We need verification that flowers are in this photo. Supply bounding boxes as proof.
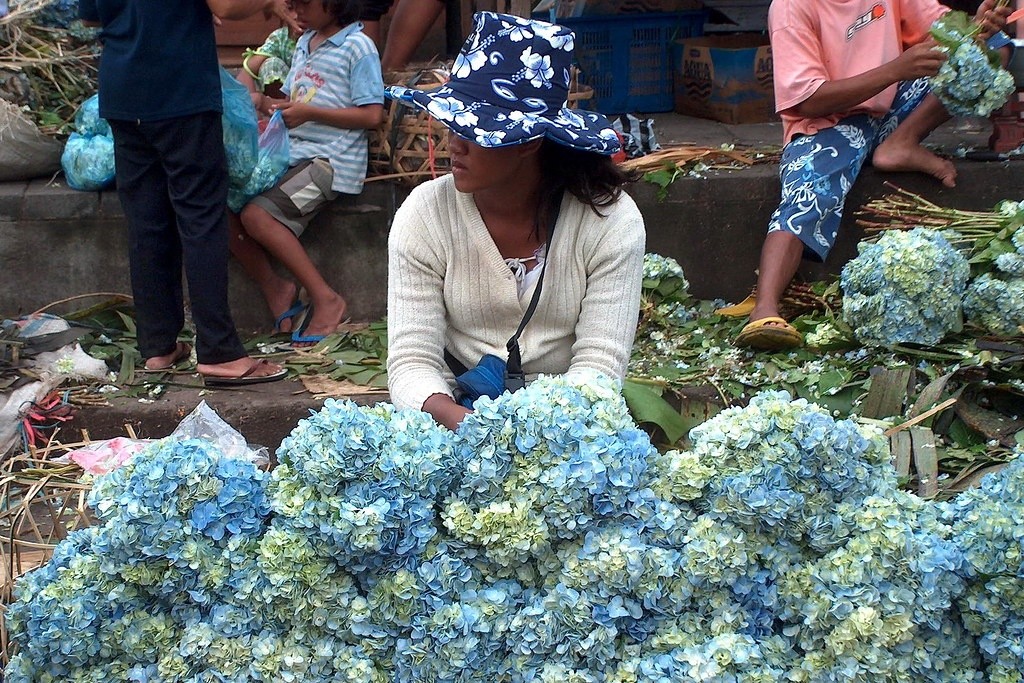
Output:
[839,225,970,347]
[962,272,1024,339]
[927,0,1016,119]
[996,200,1024,277]
[0,374,1024,683]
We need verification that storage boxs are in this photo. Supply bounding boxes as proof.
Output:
[671,32,781,125]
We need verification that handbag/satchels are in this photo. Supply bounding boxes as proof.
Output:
[456,354,526,413]
[612,113,662,159]
[61,64,290,213]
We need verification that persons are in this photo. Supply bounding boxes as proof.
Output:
[384,11,645,432]
[734,0,1017,353]
[75,0,442,388]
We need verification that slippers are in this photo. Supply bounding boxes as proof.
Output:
[144,340,191,374]
[735,317,802,352]
[274,298,314,333]
[289,328,327,351]
[714,293,757,317]
[205,359,288,386]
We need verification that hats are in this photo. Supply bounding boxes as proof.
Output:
[384,10,622,155]
